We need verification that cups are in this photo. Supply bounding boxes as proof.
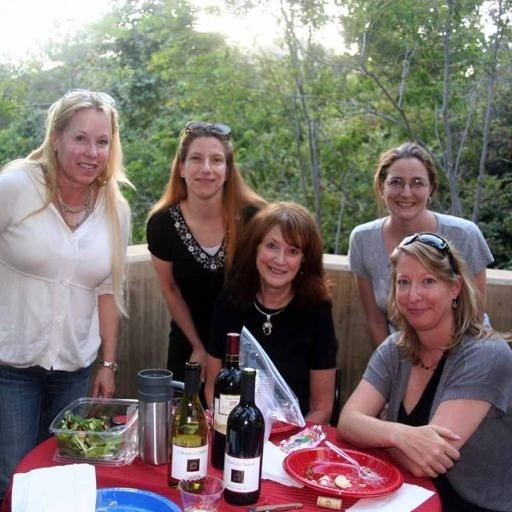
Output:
[137,368,173,465]
[178,474,226,512]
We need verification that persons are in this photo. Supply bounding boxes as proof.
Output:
[337,232,510,507]
[212,203,339,433]
[0,90,132,502]
[147,121,272,407]
[347,145,494,349]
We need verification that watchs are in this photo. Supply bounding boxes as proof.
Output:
[97,359,117,373]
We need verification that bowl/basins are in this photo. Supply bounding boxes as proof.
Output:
[49,397,139,459]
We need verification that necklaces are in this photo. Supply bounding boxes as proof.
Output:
[252,299,291,336]
[408,350,446,372]
[50,176,96,229]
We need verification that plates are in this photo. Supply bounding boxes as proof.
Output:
[281,447,404,498]
[95,487,183,512]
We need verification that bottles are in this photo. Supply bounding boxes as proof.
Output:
[167,361,209,494]
[211,333,243,470]
[223,367,266,506]
[137,368,185,466]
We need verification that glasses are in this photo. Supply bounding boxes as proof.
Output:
[183,118,232,138]
[384,175,433,193]
[399,231,461,276]
[63,88,117,106]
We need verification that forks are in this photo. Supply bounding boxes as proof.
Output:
[324,440,383,481]
